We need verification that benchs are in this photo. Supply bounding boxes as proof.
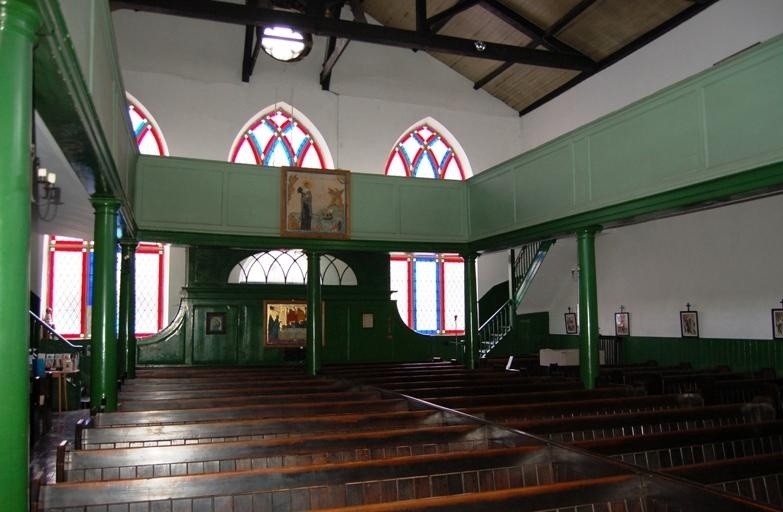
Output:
[29,361,781,511]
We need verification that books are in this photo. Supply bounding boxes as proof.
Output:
[27,352,78,372]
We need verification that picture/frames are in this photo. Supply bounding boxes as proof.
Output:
[262,299,324,347]
[563,313,577,334]
[771,308,783,341]
[205,310,226,334]
[612,312,631,337]
[679,310,699,339]
[279,166,350,238]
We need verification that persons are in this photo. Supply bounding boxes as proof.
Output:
[213,317,222,331]
[616,314,627,334]
[267,306,282,342]
[685,316,694,335]
[777,317,783,336]
[43,307,55,336]
[567,319,575,332]
[299,181,312,231]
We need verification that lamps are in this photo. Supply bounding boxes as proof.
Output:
[30,56,62,221]
